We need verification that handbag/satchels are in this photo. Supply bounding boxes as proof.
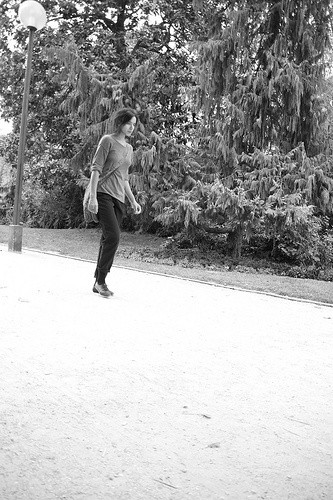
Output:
[83,183,99,223]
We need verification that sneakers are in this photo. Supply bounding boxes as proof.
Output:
[93,281,114,298]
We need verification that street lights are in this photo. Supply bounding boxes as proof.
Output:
[7,0,49,252]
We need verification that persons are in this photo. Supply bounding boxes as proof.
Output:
[88,109,142,298]
